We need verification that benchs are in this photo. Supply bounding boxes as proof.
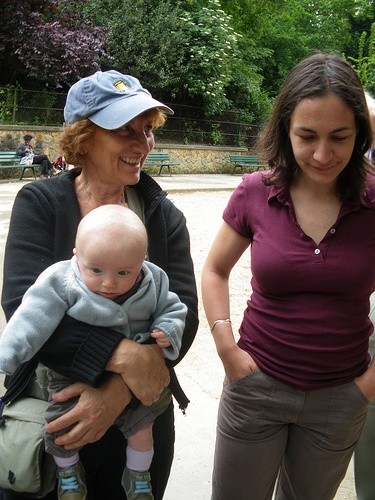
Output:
[0,151,42,180]
[229,154,269,176]
[145,153,179,177]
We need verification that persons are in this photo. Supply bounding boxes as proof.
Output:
[0,205,189,500]
[15,134,63,180]
[355,93,375,500]
[0,69,200,500]
[200,52,375,500]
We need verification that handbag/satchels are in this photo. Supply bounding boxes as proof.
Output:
[0,396,58,499]
[20,156,33,165]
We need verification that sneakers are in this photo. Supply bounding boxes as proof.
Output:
[122,466,154,500]
[55,461,88,500]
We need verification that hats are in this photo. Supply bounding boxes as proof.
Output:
[24,135,32,140]
[64,70,174,130]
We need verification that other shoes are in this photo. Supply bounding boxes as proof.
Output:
[53,170,61,175]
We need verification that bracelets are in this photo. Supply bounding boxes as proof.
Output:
[211,318,232,332]
